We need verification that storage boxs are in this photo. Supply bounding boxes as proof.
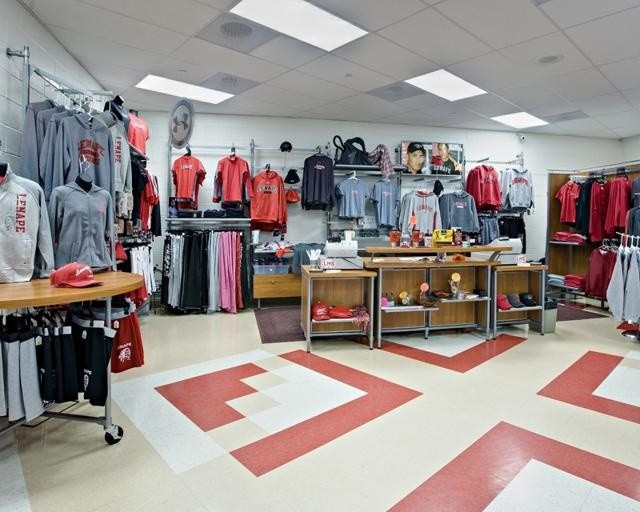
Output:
[252,263,290,274]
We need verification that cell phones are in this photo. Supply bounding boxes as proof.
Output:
[309,269,324,273]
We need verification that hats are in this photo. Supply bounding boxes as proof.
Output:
[49,261,105,288]
[407,142,425,154]
[312,300,329,320]
[280,142,292,152]
[497,292,537,309]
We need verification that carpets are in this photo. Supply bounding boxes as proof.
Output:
[251,300,612,344]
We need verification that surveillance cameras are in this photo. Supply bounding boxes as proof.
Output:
[519,136,525,140]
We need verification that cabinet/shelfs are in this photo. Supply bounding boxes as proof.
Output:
[295,246,549,353]
[253,273,302,308]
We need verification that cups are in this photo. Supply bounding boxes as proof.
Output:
[390,229,401,242]
[412,230,424,242]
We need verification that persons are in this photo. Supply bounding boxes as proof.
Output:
[403,143,463,174]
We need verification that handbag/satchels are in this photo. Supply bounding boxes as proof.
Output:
[332,136,371,165]
[284,169,300,183]
[329,305,352,318]
[286,188,301,204]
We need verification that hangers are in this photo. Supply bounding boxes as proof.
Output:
[598,238,611,250]
[617,233,640,250]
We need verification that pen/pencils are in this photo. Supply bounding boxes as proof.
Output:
[305,249,321,256]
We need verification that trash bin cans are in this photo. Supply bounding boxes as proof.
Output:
[529,297,558,332]
